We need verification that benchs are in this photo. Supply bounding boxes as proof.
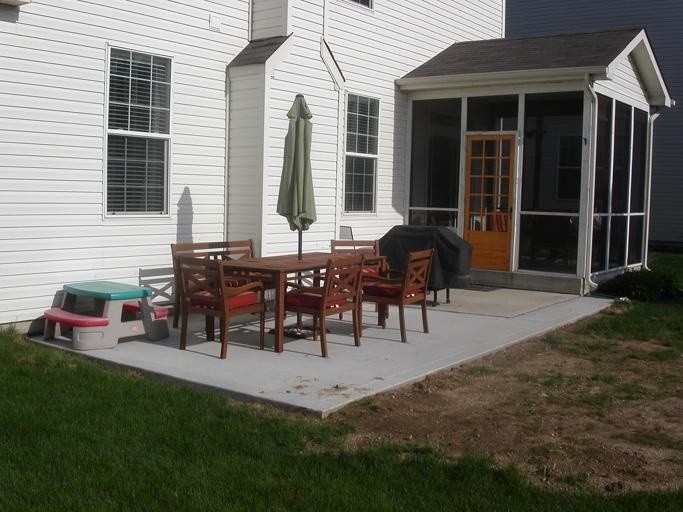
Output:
[43,301,173,350]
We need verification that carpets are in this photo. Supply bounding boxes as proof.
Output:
[404,283,580,318]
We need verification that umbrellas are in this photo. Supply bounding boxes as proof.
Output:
[276,94,317,260]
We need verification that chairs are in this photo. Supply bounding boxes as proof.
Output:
[171,239,434,358]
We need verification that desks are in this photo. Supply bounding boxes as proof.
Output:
[61,281,152,351]
